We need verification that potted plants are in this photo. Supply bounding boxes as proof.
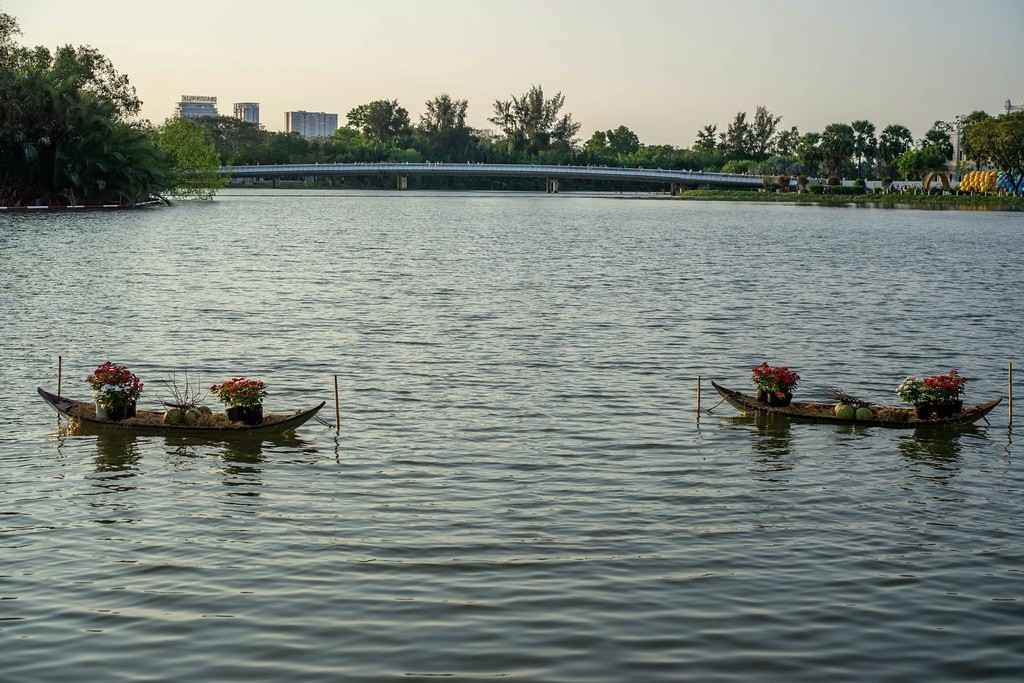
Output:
[796,177,809,194]
[776,176,790,194]
[758,175,770,193]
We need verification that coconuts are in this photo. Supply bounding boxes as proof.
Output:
[164,406,212,426]
[834,402,874,420]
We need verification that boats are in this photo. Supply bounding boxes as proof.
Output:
[37,387,326,434]
[711,381,1003,427]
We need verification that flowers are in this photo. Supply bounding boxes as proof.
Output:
[896,376,931,402]
[210,378,245,407]
[765,366,801,399]
[750,362,773,391]
[85,361,129,409]
[925,376,957,403]
[124,371,144,405]
[924,377,944,403]
[230,379,266,405]
[946,370,967,401]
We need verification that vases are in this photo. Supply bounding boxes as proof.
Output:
[123,400,137,419]
[767,392,793,408]
[952,400,963,413]
[915,402,932,420]
[224,406,244,423]
[937,404,954,419]
[932,402,939,413]
[757,387,768,401]
[243,405,264,425]
[95,401,123,422]
